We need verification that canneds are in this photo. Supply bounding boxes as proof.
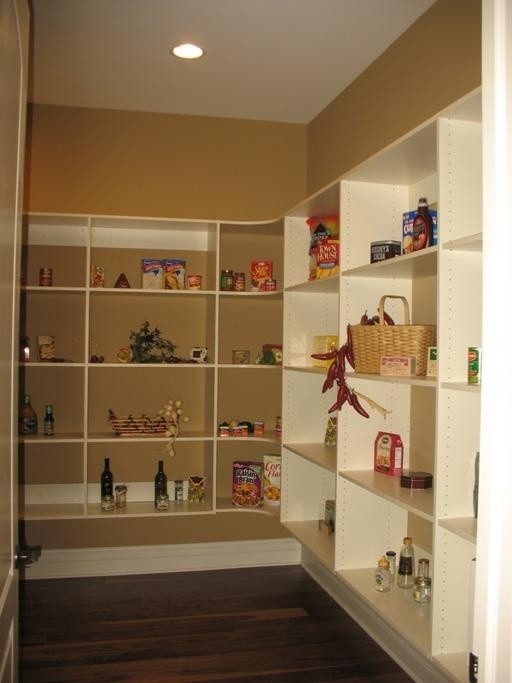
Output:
[254,421,264,436]
[275,417,282,437]
[232,425,249,437]
[468,348,481,384]
[221,270,244,291]
[39,266,53,287]
[370,241,401,264]
[187,274,202,290]
[265,279,276,291]
[230,349,250,364]
[219,427,229,436]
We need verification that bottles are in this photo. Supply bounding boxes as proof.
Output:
[45,405,55,437]
[18,394,38,436]
[373,535,432,606]
[411,197,434,253]
[221,269,234,291]
[102,493,115,512]
[154,459,168,508]
[18,335,32,363]
[274,416,282,440]
[233,272,246,292]
[37,266,53,286]
[100,457,112,502]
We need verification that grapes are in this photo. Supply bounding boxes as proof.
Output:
[90,354,105,364]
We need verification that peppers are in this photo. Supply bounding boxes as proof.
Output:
[311,306,395,418]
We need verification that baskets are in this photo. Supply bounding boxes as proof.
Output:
[106,413,181,437]
[348,294,437,377]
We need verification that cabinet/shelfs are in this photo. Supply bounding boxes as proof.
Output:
[280,87,486,683]
[10,215,284,520]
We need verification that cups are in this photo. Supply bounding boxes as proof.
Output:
[156,493,169,511]
[115,485,128,508]
